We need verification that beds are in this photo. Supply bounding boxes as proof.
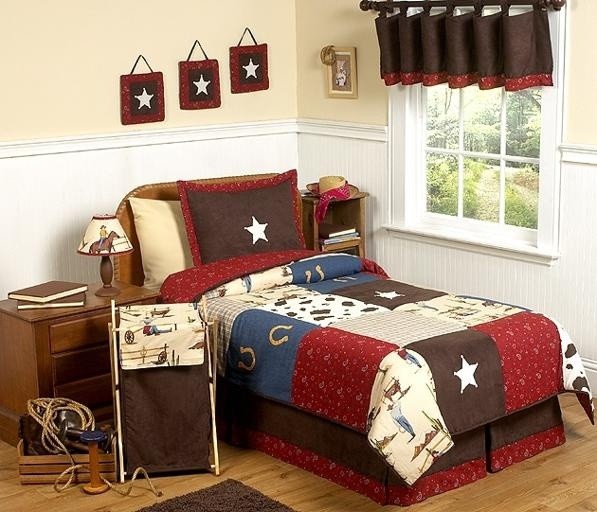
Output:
[113,174,595,506]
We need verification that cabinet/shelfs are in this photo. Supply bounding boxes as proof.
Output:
[301,191,386,260]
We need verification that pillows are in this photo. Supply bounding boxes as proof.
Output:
[127,192,193,289]
[174,168,312,267]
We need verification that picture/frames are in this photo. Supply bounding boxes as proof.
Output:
[228,43,270,95]
[319,42,358,100]
[176,57,221,111]
[115,71,166,128]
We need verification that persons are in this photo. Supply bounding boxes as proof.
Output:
[97,225,109,253]
[143,317,172,336]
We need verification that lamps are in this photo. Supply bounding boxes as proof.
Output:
[74,213,134,297]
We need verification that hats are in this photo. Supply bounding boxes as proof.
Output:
[307,175,358,200]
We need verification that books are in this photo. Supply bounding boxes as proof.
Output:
[317,223,360,245]
[7,280,88,311]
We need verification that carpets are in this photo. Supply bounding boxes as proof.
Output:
[134,476,298,512]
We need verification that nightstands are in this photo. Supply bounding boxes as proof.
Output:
[0,279,160,451]
